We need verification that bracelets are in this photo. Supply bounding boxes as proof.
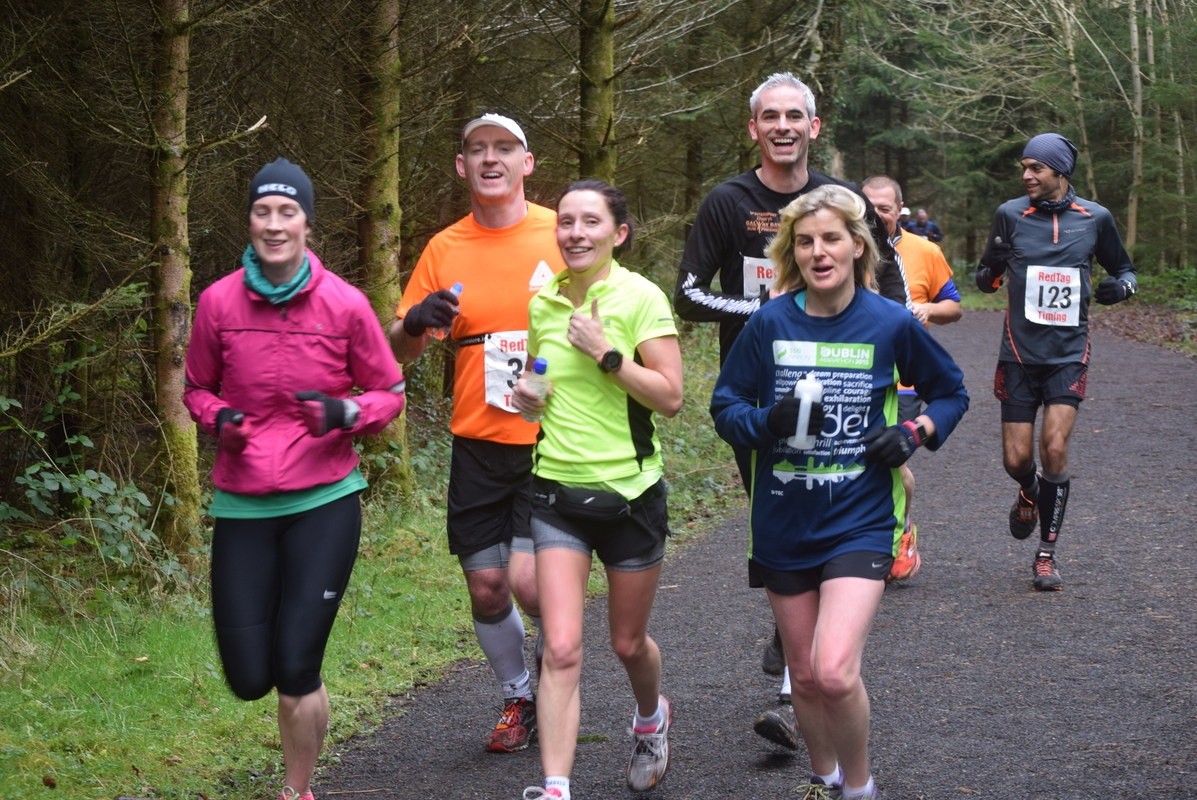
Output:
[902,421,923,449]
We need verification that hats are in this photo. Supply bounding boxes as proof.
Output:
[250,157,314,219]
[463,113,528,151]
[1022,133,1078,178]
[900,208,910,216]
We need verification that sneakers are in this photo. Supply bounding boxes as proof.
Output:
[280,787,313,800]
[761,627,785,674]
[890,523,921,580]
[753,704,801,750]
[838,783,881,800]
[1033,558,1064,590]
[487,693,537,752]
[795,768,844,800]
[522,786,570,800]
[535,630,544,660]
[626,694,673,791]
[1010,472,1043,539]
[779,667,792,701]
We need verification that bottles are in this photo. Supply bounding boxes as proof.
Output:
[786,374,823,450]
[519,357,548,423]
[425,283,463,347]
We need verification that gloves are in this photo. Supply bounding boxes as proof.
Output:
[216,409,248,454]
[987,236,1014,276]
[928,232,934,238]
[768,373,825,438]
[295,391,359,438]
[862,419,930,470]
[1094,278,1128,305]
[403,290,459,336]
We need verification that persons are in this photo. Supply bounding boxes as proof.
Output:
[675,72,911,751]
[975,130,1139,591]
[513,179,674,800]
[709,184,970,800]
[861,174,963,583]
[181,156,408,800]
[387,113,567,752]
[896,207,944,244]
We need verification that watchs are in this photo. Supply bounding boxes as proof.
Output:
[596,345,623,374]
[344,397,362,428]
[910,418,928,446]
